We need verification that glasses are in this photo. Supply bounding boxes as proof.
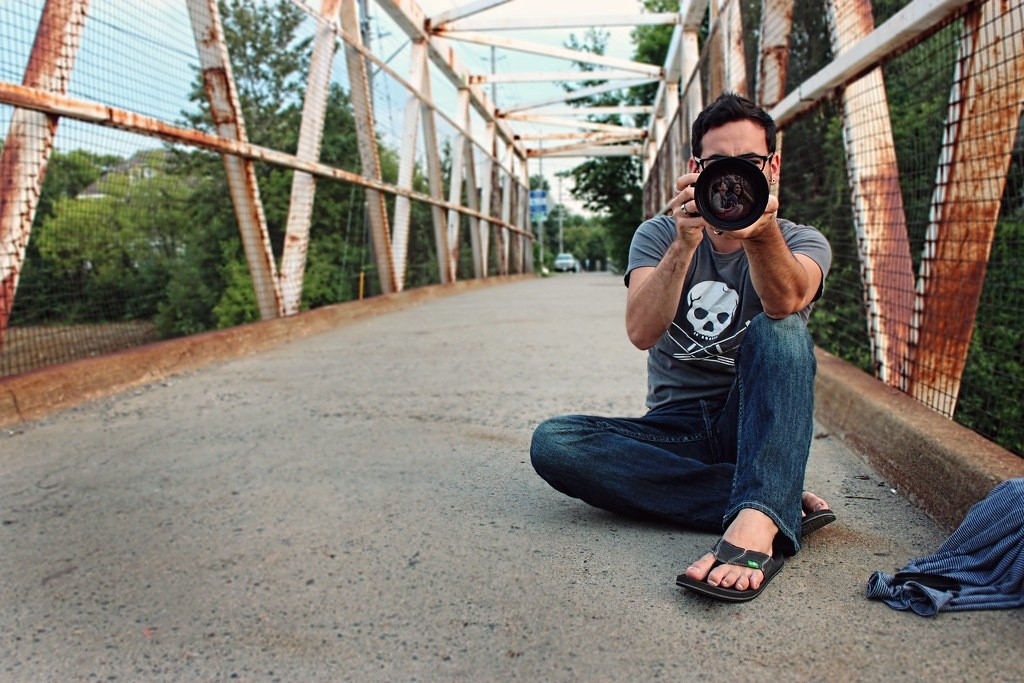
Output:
[694,153,775,171]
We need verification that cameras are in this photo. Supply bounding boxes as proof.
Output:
[687,156,769,231]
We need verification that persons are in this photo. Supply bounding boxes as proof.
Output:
[711,181,744,218]
[530,91,837,602]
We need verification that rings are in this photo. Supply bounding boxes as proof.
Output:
[713,230,724,236]
[681,204,688,214]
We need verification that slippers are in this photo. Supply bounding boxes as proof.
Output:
[676,538,785,602]
[802,490,836,538]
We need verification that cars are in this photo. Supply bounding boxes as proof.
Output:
[554,253,580,273]
